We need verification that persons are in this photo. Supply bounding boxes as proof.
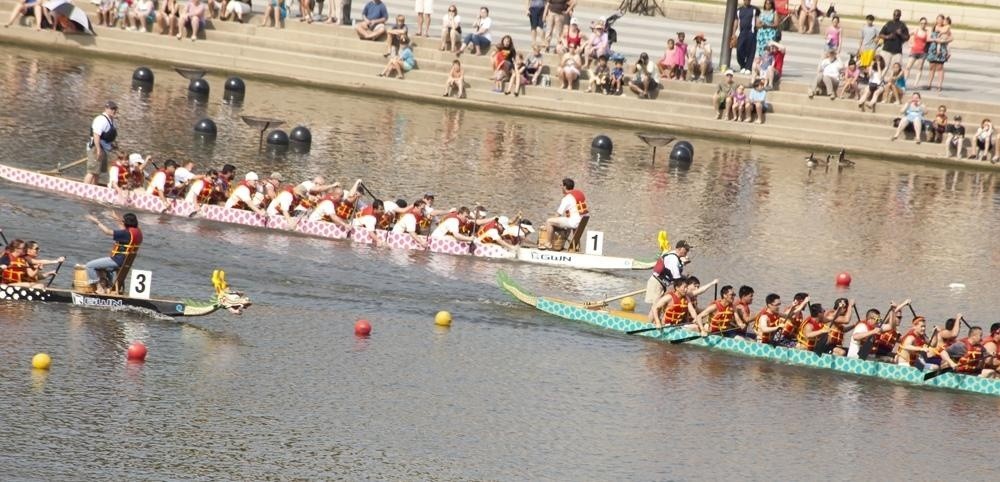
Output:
[105,153,534,253]
[732,1,952,109]
[259,0,388,40]
[84,212,142,297]
[491,1,660,98]
[646,241,691,323]
[378,0,491,99]
[1,239,24,284]
[2,1,253,40]
[892,93,994,160]
[657,31,712,82]
[86,101,119,185]
[25,243,67,281]
[651,275,1000,377]
[538,178,588,251]
[713,70,768,124]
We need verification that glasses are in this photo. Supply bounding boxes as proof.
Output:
[209,175,218,181]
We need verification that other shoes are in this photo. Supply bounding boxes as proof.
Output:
[440,45,483,57]
[715,111,762,124]
[300,17,345,25]
[379,52,403,78]
[661,71,707,83]
[424,33,428,37]
[493,77,650,98]
[809,75,941,144]
[457,96,462,98]
[443,93,448,97]
[741,68,773,91]
[416,32,420,35]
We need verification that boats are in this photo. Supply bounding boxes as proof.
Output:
[0,269,253,319]
[494,266,999,397]
[0,155,661,274]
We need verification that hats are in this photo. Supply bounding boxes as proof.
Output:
[271,171,286,182]
[725,69,733,76]
[129,154,145,163]
[520,223,535,232]
[105,101,118,109]
[400,38,409,43]
[165,159,180,168]
[293,184,308,199]
[499,216,509,231]
[676,240,693,249]
[244,171,259,181]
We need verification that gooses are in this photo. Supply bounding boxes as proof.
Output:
[837,147,856,167]
[817,153,835,167]
[804,151,817,168]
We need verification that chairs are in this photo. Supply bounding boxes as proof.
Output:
[103,251,136,294]
[564,214,590,251]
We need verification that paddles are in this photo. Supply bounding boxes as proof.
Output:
[626,323,695,335]
[961,316,971,329]
[385,207,525,261]
[585,282,717,309]
[138,158,223,219]
[774,301,929,362]
[924,354,992,381]
[258,178,380,241]
[670,326,741,345]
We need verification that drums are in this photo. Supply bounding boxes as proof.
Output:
[74,265,98,296]
[539,226,567,252]
